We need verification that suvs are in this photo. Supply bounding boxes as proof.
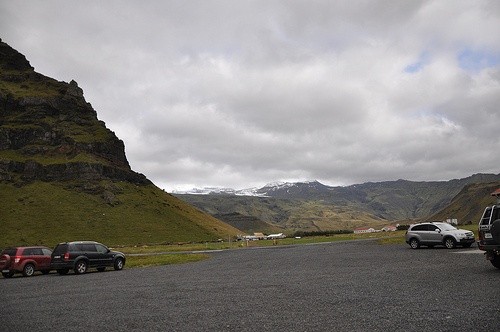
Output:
[48,240,126,275]
[0,246,54,278]
[405,221,475,250]
[478,204,500,270]
